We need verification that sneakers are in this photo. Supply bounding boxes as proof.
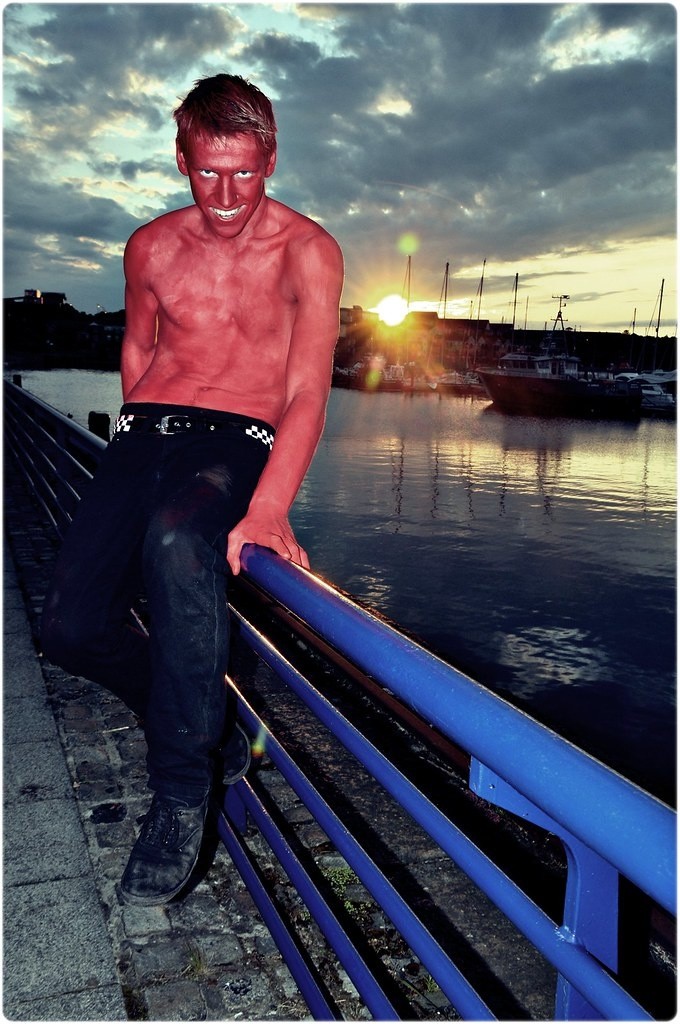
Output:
[215,717,250,788]
[119,795,207,910]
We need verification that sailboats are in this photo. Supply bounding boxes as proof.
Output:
[333,256,676,419]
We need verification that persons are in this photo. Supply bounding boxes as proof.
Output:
[61,68,344,907]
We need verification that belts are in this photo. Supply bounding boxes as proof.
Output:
[112,414,277,454]
